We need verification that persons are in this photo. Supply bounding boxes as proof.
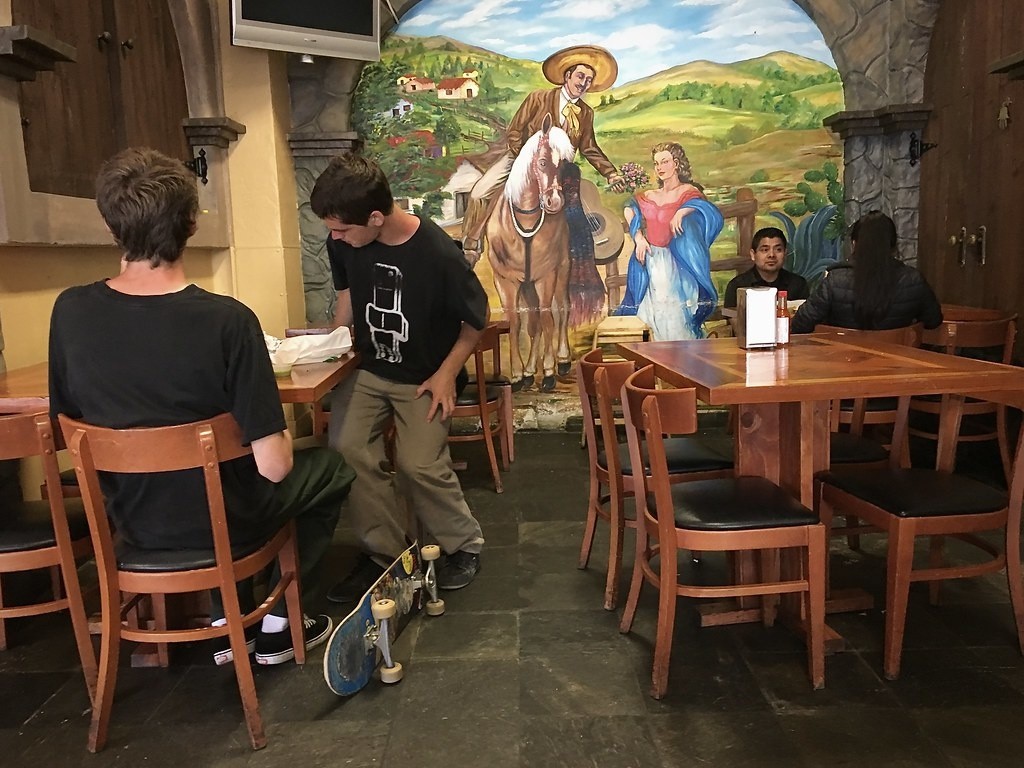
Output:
[791,209,946,335]
[723,226,813,342]
[311,147,492,609]
[48,149,356,668]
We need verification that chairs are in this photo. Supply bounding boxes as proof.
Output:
[617,363,828,699]
[387,322,509,495]
[572,349,733,612]
[900,313,1018,487]
[53,412,307,754]
[814,324,922,550]
[462,322,516,471]
[814,397,1023,683]
[1,405,144,714]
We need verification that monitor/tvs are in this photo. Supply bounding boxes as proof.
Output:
[229,0,380,62]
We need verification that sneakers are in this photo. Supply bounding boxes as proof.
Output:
[211,612,258,665]
[438,549,481,589]
[255,615,333,665]
[327,562,384,603]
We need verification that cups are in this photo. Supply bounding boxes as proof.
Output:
[272,364,293,378]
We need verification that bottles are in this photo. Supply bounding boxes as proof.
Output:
[775,291,792,347]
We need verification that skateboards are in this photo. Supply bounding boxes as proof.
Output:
[322,536,447,697]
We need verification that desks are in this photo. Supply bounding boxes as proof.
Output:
[285,327,459,475]
[720,303,1004,337]
[0,338,360,635]
[616,331,1024,628]
